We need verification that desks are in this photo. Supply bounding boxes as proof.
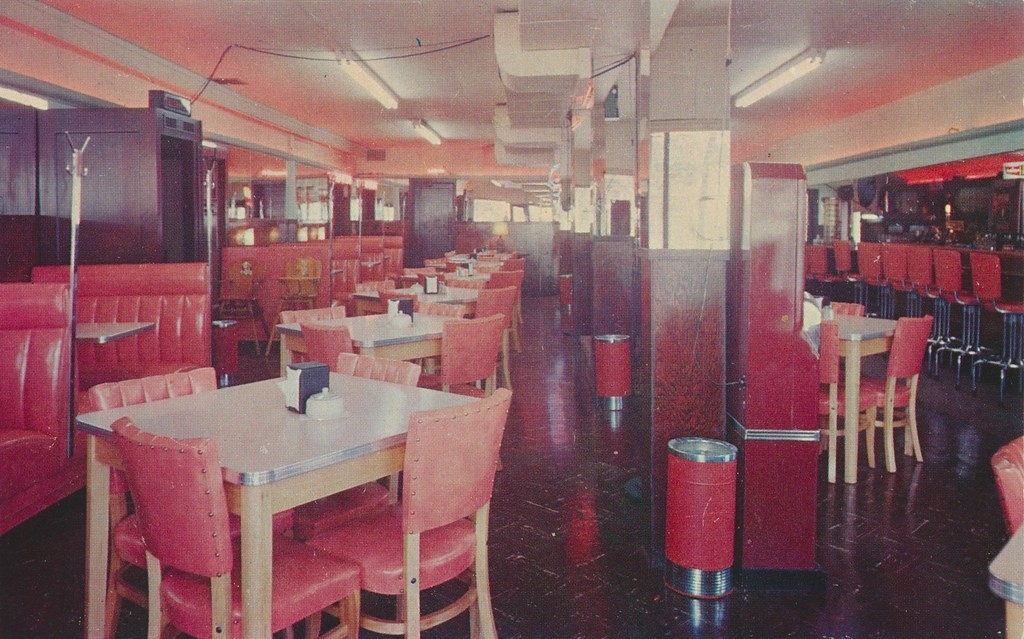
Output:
[400,273,490,289]
[448,253,512,263]
[75,370,488,639]
[988,525,1024,639]
[427,261,504,274]
[837,315,899,483]
[352,287,486,313]
[275,311,468,378]
[72,322,155,344]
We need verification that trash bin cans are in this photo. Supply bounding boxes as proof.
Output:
[594,335,631,411]
[664,436,738,599]
[559,275,573,335]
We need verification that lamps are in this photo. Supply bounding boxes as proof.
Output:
[335,49,398,110]
[730,46,827,109]
[412,120,442,146]
[492,222,509,254]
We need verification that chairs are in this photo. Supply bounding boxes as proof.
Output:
[87,246,529,639]
[809,301,1024,538]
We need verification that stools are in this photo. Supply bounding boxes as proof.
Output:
[805,233,1024,405]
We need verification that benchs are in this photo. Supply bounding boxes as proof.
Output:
[221,231,405,342]
[1,236,213,534]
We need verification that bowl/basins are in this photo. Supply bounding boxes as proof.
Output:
[410,283,424,294]
[389,310,413,329]
[304,387,348,420]
